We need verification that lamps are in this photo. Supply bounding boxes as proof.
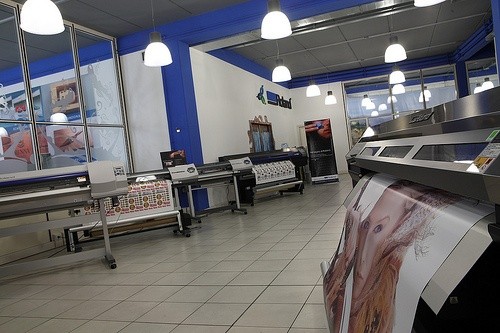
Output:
[272,40,292,82]
[325,72,337,105]
[474,65,484,94]
[144,0,173,67]
[261,0,292,40]
[482,69,494,90]
[385,14,407,63]
[19,0,65,35]
[306,80,321,98]
[362,63,431,117]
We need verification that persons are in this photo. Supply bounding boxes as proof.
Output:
[304,118,331,138]
[323,179,461,333]
